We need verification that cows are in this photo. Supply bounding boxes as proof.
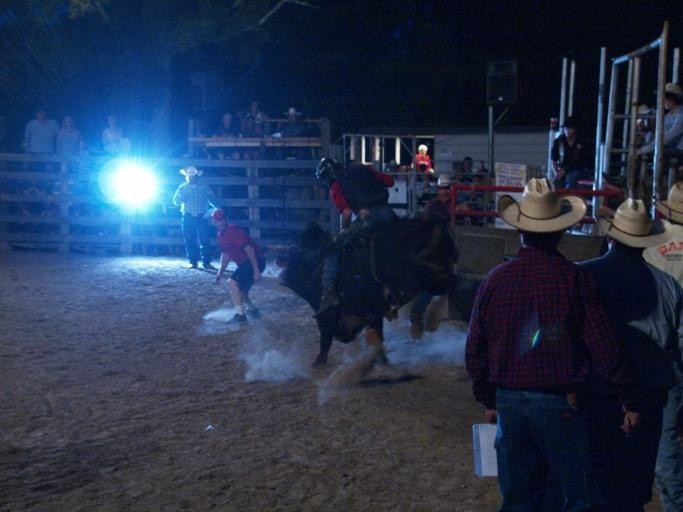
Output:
[275,213,462,372]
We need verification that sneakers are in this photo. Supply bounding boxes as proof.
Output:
[223,314,247,325]
[188,262,213,270]
[245,308,260,320]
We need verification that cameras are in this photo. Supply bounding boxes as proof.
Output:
[550,175,566,184]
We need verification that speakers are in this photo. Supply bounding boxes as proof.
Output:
[485,59,518,106]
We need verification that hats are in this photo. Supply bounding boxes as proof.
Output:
[179,166,204,176]
[496,177,588,233]
[428,174,457,187]
[283,107,303,116]
[654,181,683,224]
[596,197,672,249]
[561,116,579,128]
[214,212,226,221]
[636,104,652,114]
[653,82,682,96]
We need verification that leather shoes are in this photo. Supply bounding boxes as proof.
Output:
[311,289,341,318]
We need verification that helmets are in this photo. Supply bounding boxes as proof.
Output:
[315,156,342,184]
